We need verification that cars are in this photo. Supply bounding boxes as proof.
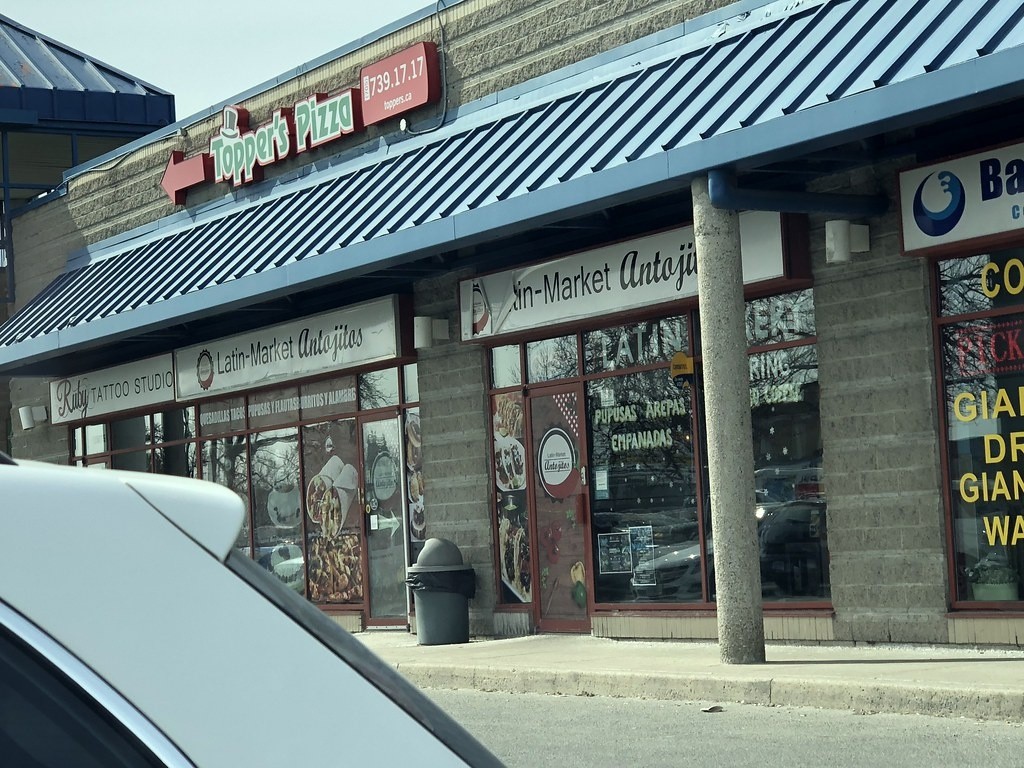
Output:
[0,452,505,768]
[635,467,823,601]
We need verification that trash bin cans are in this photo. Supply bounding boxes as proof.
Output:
[406,538,474,644]
